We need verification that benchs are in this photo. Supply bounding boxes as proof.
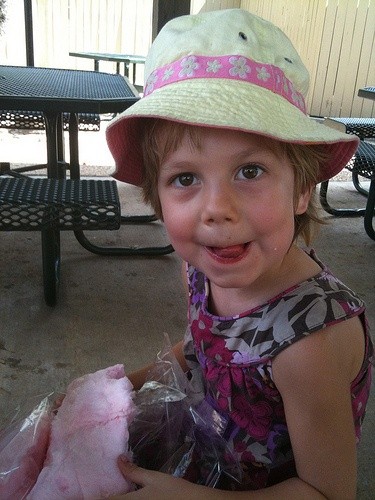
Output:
[0,178,121,306]
[325,117,375,183]
[345,140,375,226]
[0,110,100,132]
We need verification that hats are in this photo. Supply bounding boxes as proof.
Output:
[105,8,360,189]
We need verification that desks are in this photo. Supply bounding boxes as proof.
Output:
[0,64,142,179]
[69,52,146,85]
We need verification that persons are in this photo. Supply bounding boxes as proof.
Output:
[55,8,375,500]
